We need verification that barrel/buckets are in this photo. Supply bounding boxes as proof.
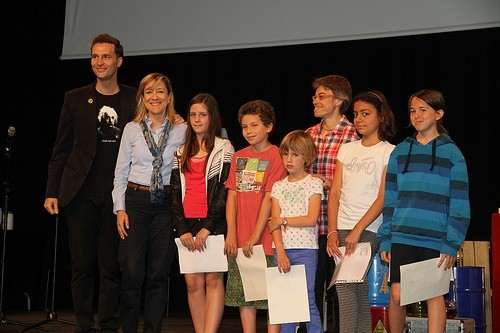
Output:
[445,266,486,333]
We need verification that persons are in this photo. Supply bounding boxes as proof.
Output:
[169,92,236,333]
[377,90,470,333]
[44,35,139,333]
[326,93,397,333]
[299,76,360,333]
[224,100,287,333]
[270,129,328,333]
[111,73,188,333]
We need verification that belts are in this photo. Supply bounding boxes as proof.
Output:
[127,182,171,193]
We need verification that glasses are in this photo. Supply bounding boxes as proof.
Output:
[312,93,333,101]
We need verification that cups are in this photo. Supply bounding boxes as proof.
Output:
[446,299,457,320]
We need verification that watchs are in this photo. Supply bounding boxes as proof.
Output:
[282,217,287,228]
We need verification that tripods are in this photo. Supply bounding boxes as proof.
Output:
[0,151,76,333]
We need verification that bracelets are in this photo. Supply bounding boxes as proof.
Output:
[327,230,338,240]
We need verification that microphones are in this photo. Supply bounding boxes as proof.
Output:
[5,126,15,151]
